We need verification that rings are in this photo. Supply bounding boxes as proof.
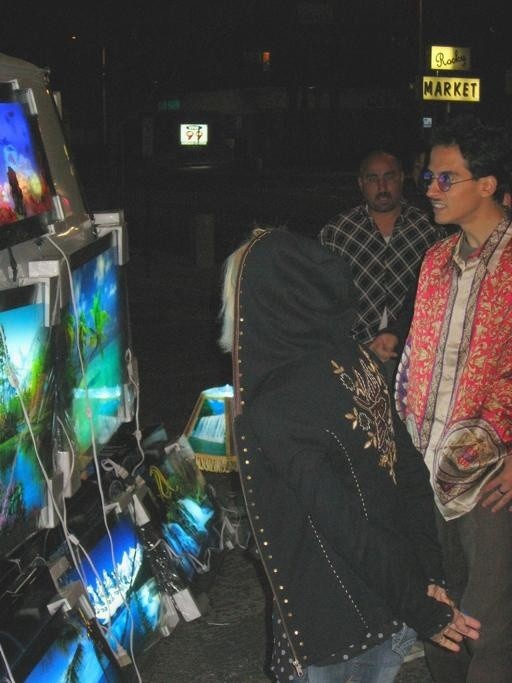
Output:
[498,489,505,497]
[440,639,443,645]
[444,628,451,637]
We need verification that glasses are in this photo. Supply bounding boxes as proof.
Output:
[423,170,473,192]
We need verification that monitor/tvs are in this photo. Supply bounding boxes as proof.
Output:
[178,121,212,148]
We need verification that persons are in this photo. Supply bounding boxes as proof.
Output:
[315,147,448,344]
[367,166,512,360]
[216,225,482,683]
[392,120,512,682]
